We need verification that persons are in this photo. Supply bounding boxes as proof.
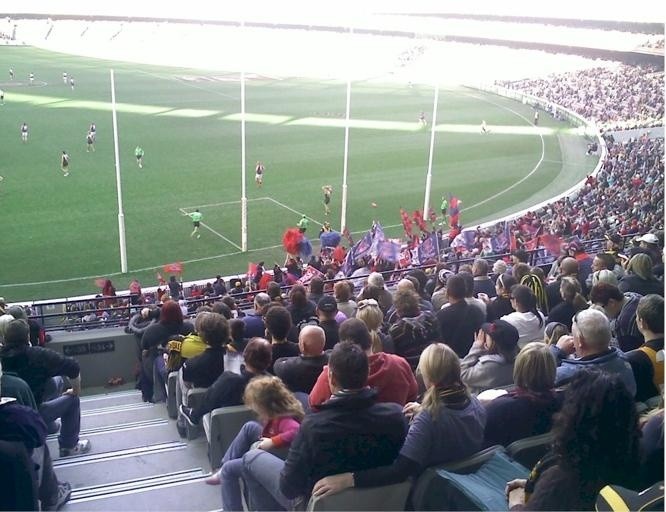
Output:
[481,120,487,135]
[86,124,96,152]
[418,111,428,127]
[124,132,665,512]
[61,151,71,177]
[9,67,76,90]
[494,32,663,132]
[66,279,118,328]
[21,123,28,142]
[1,16,19,47]
[1,296,90,512]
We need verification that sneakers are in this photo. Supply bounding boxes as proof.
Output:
[58,439,91,457]
[206,472,221,486]
[41,479,72,512]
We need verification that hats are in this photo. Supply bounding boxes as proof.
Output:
[635,233,659,245]
[481,320,520,347]
[317,295,337,313]
